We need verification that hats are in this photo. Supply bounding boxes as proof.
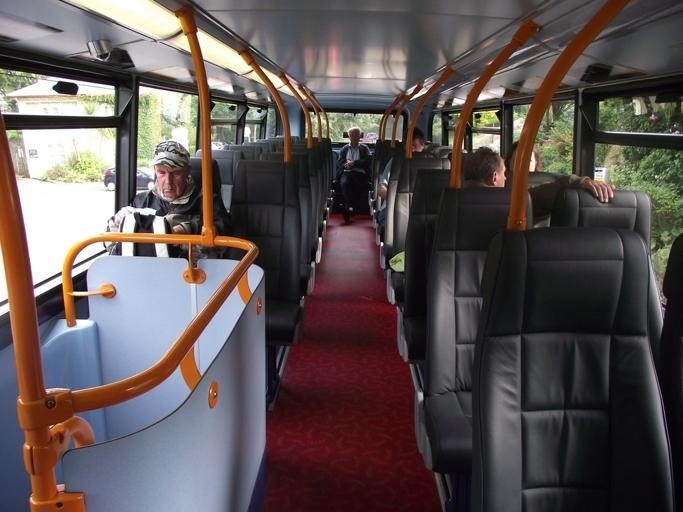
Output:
[149,140,190,170]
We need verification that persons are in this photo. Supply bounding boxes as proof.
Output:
[376,126,424,241]
[503,140,543,173]
[460,146,616,227]
[102,139,233,259]
[335,127,373,224]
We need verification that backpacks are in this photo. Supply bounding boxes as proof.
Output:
[108,206,183,258]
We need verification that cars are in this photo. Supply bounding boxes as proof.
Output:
[104,163,156,192]
